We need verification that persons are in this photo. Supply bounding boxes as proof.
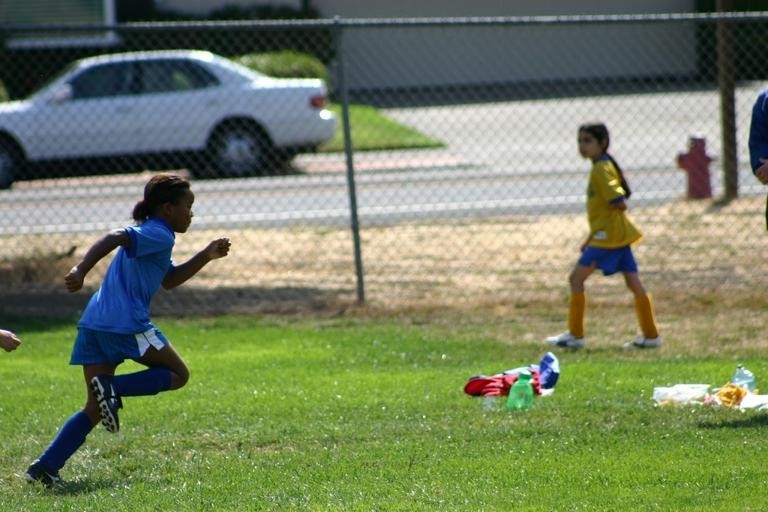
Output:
[27,175,231,489]
[544,124,661,350]
[749,88,768,230]
[0,329,21,352]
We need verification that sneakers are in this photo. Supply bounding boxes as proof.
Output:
[25,462,60,488]
[89,375,120,433]
[625,336,661,350]
[546,331,584,348]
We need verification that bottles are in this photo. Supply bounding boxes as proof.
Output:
[508,373,532,414]
[735,363,756,394]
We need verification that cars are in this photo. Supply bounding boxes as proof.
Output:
[0,48,338,189]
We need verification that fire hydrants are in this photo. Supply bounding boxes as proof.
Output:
[677,135,715,201]
